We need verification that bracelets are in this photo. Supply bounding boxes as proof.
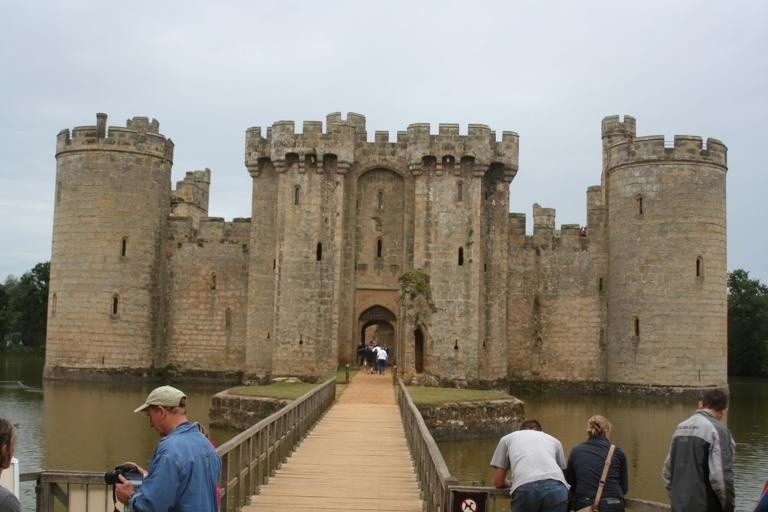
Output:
[126,493,133,504]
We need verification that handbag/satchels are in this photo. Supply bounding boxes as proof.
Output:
[570,505,598,512]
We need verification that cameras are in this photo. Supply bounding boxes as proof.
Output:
[104,465,143,488]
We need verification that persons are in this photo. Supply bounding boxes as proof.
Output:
[200,428,222,509]
[358,339,390,375]
[489,420,572,511]
[114,386,223,512]
[662,389,735,510]
[564,414,629,512]
[0,417,23,512]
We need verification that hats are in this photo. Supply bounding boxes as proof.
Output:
[133,385,188,413]
